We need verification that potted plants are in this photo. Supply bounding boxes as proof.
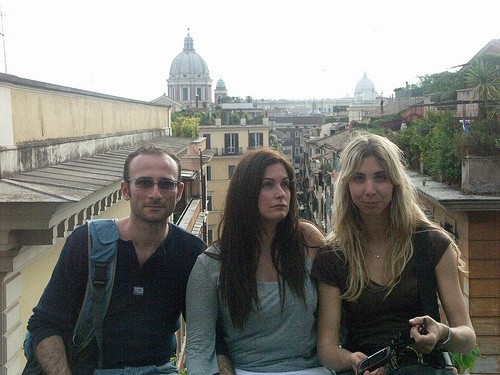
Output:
[394,108,500,194]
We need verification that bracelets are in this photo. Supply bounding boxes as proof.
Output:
[436,326,451,347]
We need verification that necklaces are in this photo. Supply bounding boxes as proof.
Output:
[368,246,387,258]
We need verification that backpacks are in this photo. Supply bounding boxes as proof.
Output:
[21,218,117,375]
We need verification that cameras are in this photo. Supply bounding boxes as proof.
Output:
[357,346,392,375]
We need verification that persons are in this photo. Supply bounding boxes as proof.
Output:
[310,132,476,375]
[294,176,334,220]
[185,148,337,375]
[21,144,236,375]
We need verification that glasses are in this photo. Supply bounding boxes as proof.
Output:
[126,176,180,192]
[389,318,426,353]
